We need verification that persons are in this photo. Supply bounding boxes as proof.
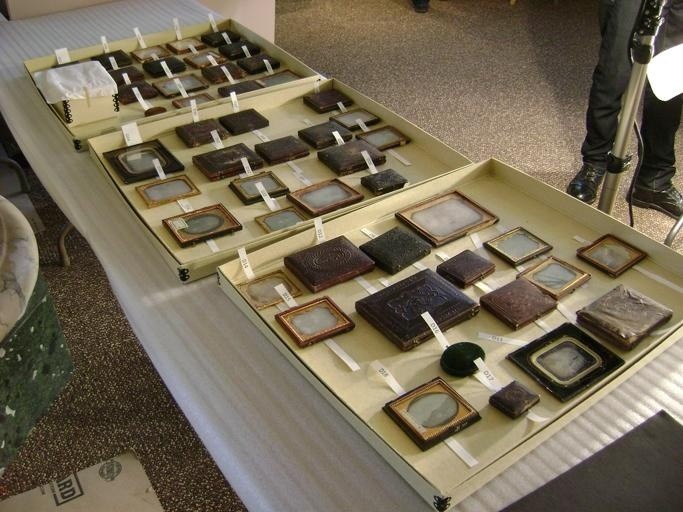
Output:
[565,1,682,221]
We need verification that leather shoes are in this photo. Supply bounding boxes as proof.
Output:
[628,184,683,220]
[567,163,605,205]
